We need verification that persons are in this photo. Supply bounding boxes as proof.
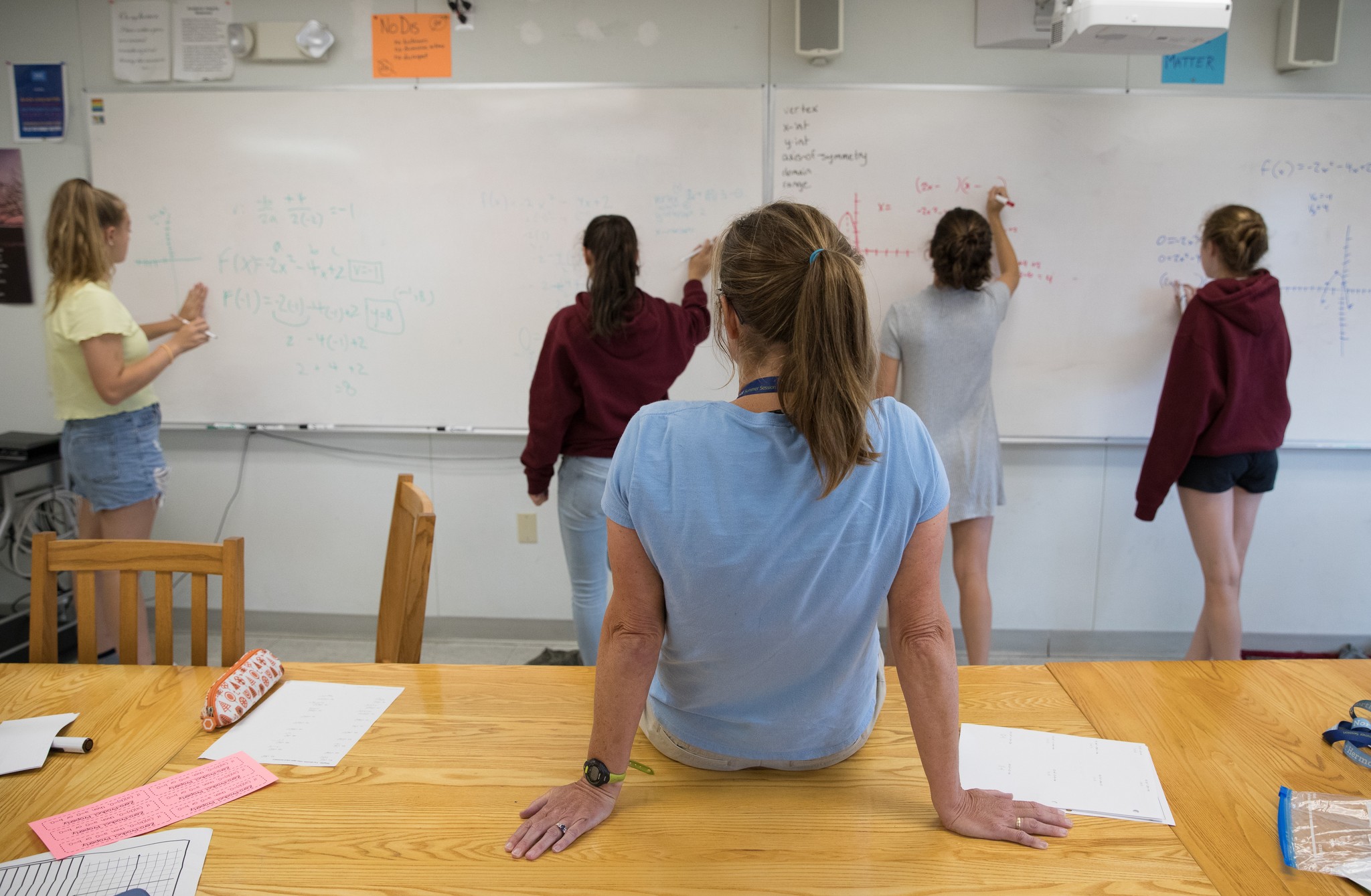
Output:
[502,201,1076,864]
[873,184,1022,664]
[1133,203,1295,661]
[517,213,716,665]
[38,178,214,663]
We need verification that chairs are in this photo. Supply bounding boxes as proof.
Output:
[30,531,245,667]
[375,474,436,663]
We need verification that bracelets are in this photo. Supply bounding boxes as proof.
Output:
[160,343,174,365]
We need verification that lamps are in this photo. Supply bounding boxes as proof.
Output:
[226,17,334,67]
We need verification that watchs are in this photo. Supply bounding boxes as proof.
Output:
[583,758,655,787]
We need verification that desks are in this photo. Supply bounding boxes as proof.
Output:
[1047,658,1371,896]
[0,662,233,896]
[110,664,1220,896]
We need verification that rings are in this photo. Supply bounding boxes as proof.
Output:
[555,823,568,834]
[1016,817,1021,831]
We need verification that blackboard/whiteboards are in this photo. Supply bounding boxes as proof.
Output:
[85,87,767,436]
[767,79,1371,446]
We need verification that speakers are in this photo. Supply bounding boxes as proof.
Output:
[795,0,844,60]
[1277,0,1344,68]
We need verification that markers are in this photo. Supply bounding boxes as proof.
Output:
[169,312,218,341]
[1179,284,1187,316]
[49,735,94,756]
[298,423,336,431]
[679,241,715,265]
[437,425,472,434]
[206,422,246,430]
[994,193,1015,209]
[247,424,285,432]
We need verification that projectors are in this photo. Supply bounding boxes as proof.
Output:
[1049,1,1233,56]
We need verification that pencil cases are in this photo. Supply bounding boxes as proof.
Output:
[196,648,286,736]
